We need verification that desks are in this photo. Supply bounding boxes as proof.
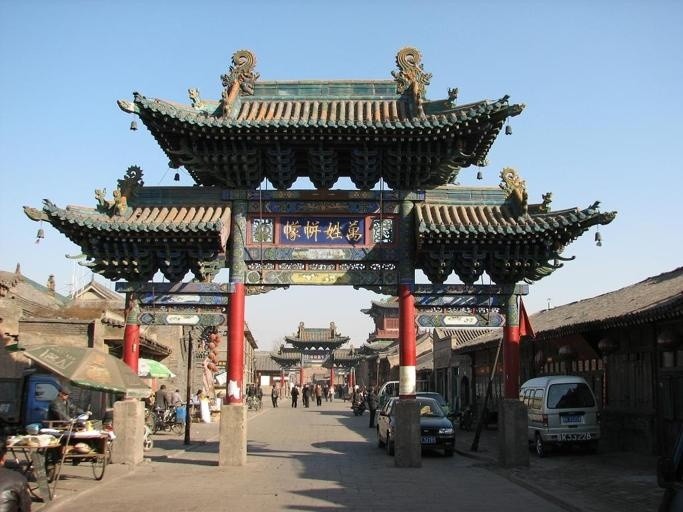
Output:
[5,442,62,504]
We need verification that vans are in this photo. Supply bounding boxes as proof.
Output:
[378,379,428,408]
[518,376,601,457]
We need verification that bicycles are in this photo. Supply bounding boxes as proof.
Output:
[246,394,263,410]
[144,406,182,434]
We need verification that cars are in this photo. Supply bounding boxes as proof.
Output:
[416,391,449,415]
[376,396,455,457]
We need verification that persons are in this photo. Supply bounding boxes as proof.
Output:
[1,428,31,512]
[47,386,92,427]
[141,385,182,428]
[249,383,379,428]
[195,390,203,401]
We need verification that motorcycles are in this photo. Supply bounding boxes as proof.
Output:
[106,420,154,452]
[351,396,366,416]
[458,406,473,432]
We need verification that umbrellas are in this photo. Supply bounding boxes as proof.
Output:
[21,344,153,500]
[138,358,176,379]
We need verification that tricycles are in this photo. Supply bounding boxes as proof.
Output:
[39,402,108,482]
